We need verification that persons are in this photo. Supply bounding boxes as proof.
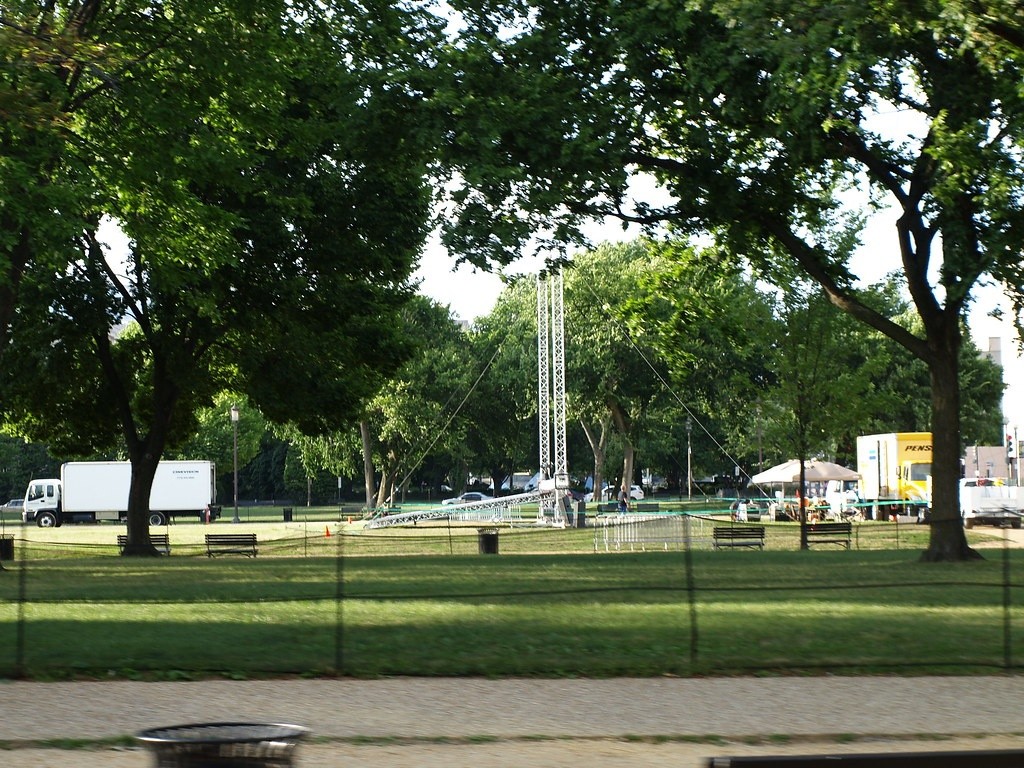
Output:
[819,487,823,497]
[618,485,628,514]
[470,474,475,485]
[820,500,828,520]
[852,486,858,494]
[730,498,742,522]
[784,505,797,522]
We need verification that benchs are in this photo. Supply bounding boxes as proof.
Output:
[799,523,852,549]
[368,508,401,518]
[828,539,830,539]
[340,507,364,520]
[204,533,258,558]
[712,526,765,551]
[637,503,659,512]
[597,505,617,514]
[117,534,171,556]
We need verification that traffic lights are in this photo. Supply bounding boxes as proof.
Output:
[1006,435,1012,453]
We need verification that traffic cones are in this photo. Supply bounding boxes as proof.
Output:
[349,517,353,524]
[324,526,330,536]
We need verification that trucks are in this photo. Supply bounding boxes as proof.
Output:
[857,431,933,520]
[20,461,217,527]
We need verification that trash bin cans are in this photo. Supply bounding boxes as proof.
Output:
[131,720,314,768]
[477,528,499,555]
[0,534,14,561]
[283,507,293,521]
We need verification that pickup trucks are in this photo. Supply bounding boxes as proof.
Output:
[959,478,1024,529]
[692,476,742,492]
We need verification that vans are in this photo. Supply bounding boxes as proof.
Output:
[826,479,858,521]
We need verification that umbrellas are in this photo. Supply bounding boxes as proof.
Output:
[752,457,860,498]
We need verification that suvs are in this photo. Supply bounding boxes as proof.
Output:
[583,485,645,503]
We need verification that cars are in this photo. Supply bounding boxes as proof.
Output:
[0,500,24,513]
[442,493,494,507]
[530,488,571,503]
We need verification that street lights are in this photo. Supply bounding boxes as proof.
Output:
[231,402,241,523]
[686,419,694,502]
[756,403,762,503]
